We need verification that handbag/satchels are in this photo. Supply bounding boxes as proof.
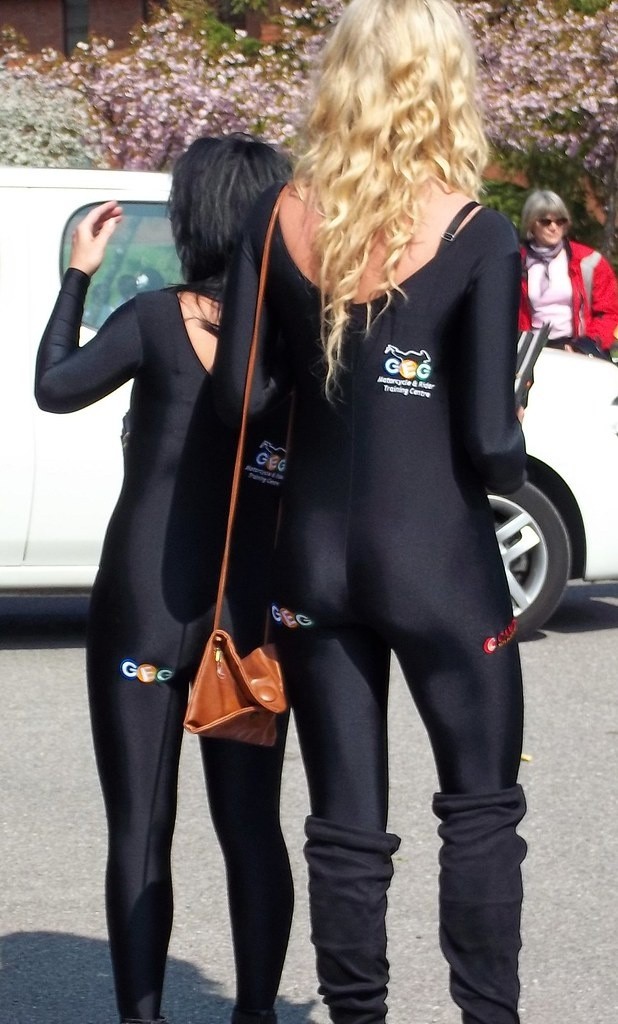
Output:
[182,630,288,746]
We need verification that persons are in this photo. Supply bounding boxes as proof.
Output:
[219,0,532,1024]
[515,189,618,365]
[33,133,297,1024]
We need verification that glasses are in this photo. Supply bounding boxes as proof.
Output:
[539,217,567,226]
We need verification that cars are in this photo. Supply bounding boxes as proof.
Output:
[1,162,618,643]
